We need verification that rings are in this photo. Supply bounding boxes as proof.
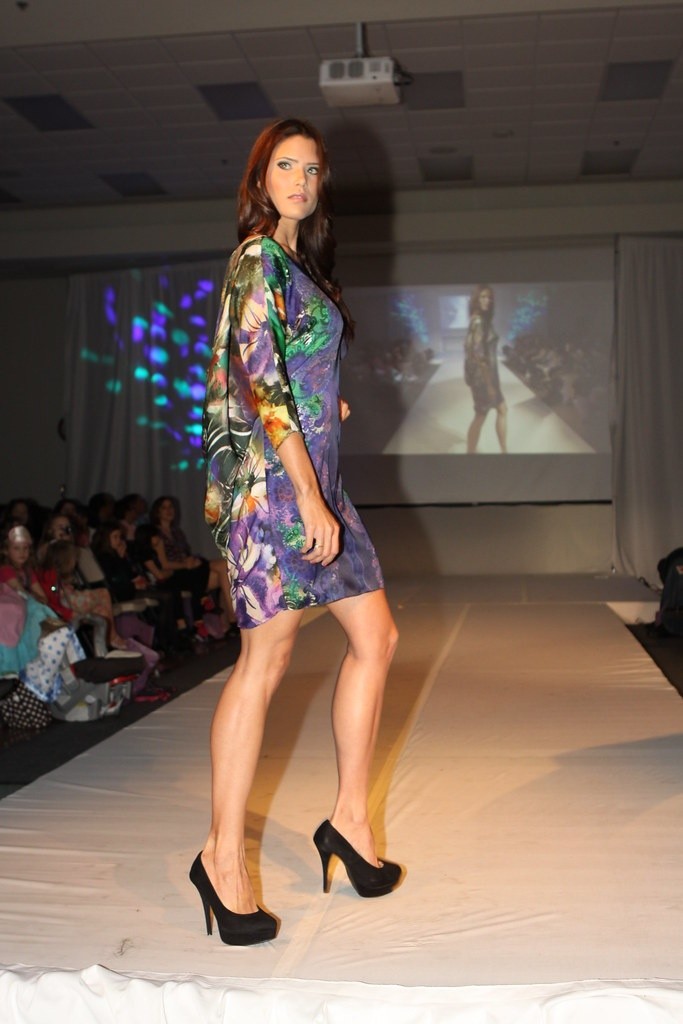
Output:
[315,545,323,548]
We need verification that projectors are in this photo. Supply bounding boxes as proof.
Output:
[318,56,401,107]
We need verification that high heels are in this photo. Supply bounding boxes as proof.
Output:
[189,851,277,946]
[312,819,403,898]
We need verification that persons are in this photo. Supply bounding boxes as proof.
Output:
[462,285,508,452]
[189,117,400,947]
[0,494,236,722]
[502,332,610,411]
[341,338,434,398]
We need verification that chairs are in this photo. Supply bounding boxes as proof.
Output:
[47,540,159,654]
